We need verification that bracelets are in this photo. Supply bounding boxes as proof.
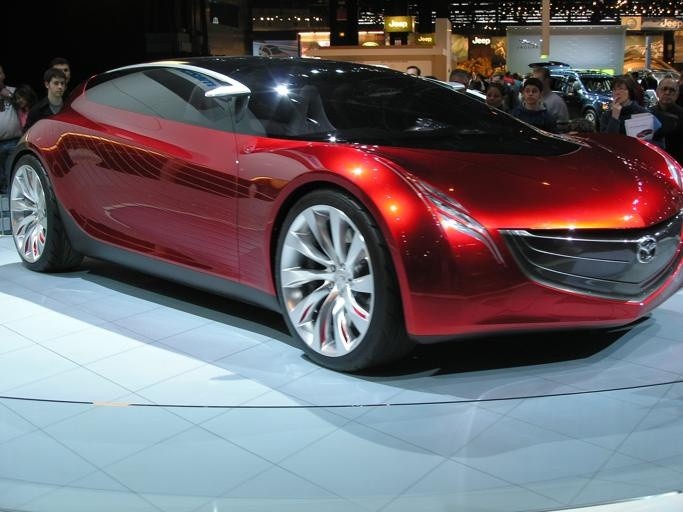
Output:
[7,90,12,100]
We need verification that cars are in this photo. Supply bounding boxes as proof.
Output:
[549,67,614,132]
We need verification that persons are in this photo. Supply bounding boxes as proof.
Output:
[19,66,70,135]
[12,83,38,130]
[405,65,421,76]
[46,55,72,106]
[449,53,682,167]
[0,66,23,193]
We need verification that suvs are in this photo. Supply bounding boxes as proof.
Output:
[259,44,289,57]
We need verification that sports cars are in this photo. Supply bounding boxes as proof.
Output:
[8,55,683,373]
